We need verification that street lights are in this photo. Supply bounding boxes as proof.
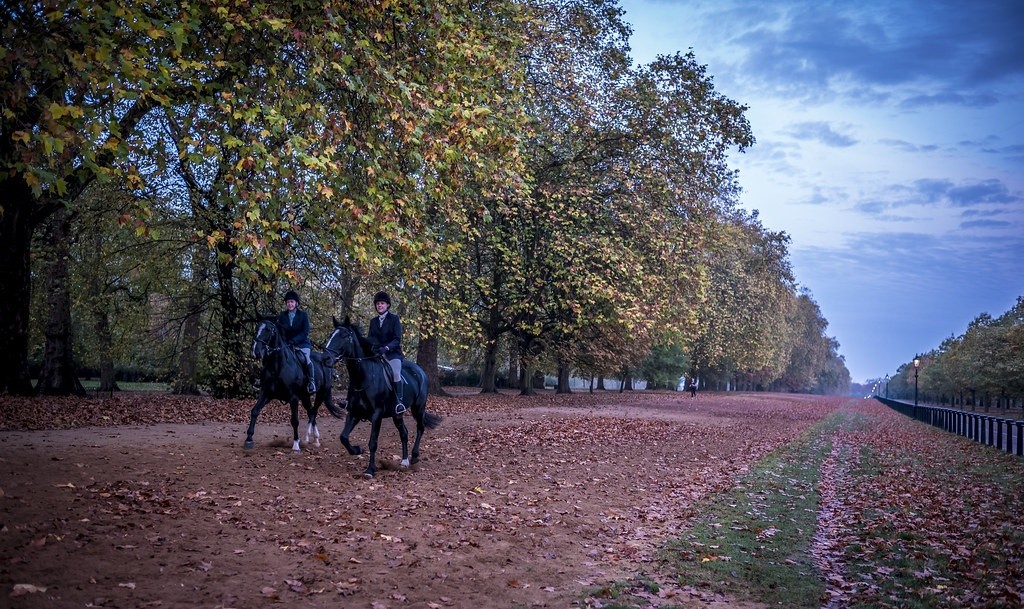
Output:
[885,374,889,398]
[913,353,921,406]
[878,379,880,396]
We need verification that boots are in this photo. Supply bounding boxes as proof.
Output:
[394,380,404,411]
[307,364,316,392]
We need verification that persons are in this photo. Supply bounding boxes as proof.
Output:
[275,291,316,394]
[336,292,406,415]
[689,377,696,397]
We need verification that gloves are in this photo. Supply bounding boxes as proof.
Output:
[374,347,386,355]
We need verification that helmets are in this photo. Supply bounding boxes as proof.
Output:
[284,291,299,302]
[374,291,392,308]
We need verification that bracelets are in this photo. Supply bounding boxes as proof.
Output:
[384,345,389,351]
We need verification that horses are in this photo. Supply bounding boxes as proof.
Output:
[320,313,445,481]
[242,310,347,452]
